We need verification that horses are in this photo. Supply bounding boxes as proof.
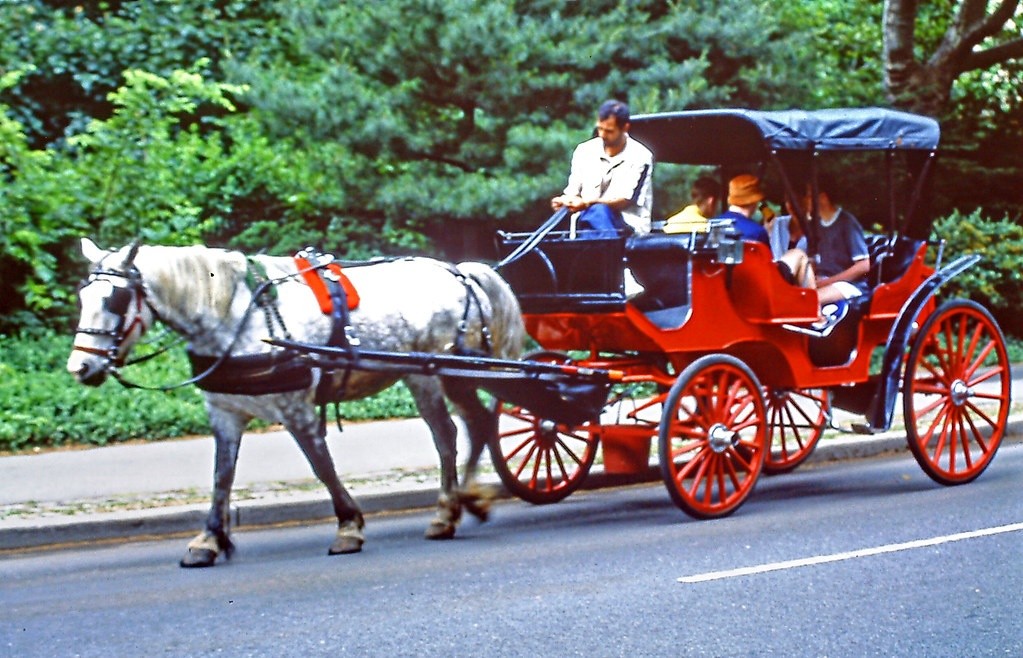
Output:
[66,235,528,568]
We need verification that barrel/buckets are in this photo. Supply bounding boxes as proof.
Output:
[601,393,658,475]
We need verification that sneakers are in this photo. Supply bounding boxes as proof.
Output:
[811,301,849,338]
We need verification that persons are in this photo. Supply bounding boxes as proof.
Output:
[716,175,830,330]
[769,182,869,304]
[662,175,720,236]
[552,100,652,238]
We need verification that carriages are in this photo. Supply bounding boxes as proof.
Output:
[65,109,1012,567]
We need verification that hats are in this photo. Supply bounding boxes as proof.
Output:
[726,174,768,206]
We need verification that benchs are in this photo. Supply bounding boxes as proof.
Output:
[624,225,820,329]
[817,233,929,355]
